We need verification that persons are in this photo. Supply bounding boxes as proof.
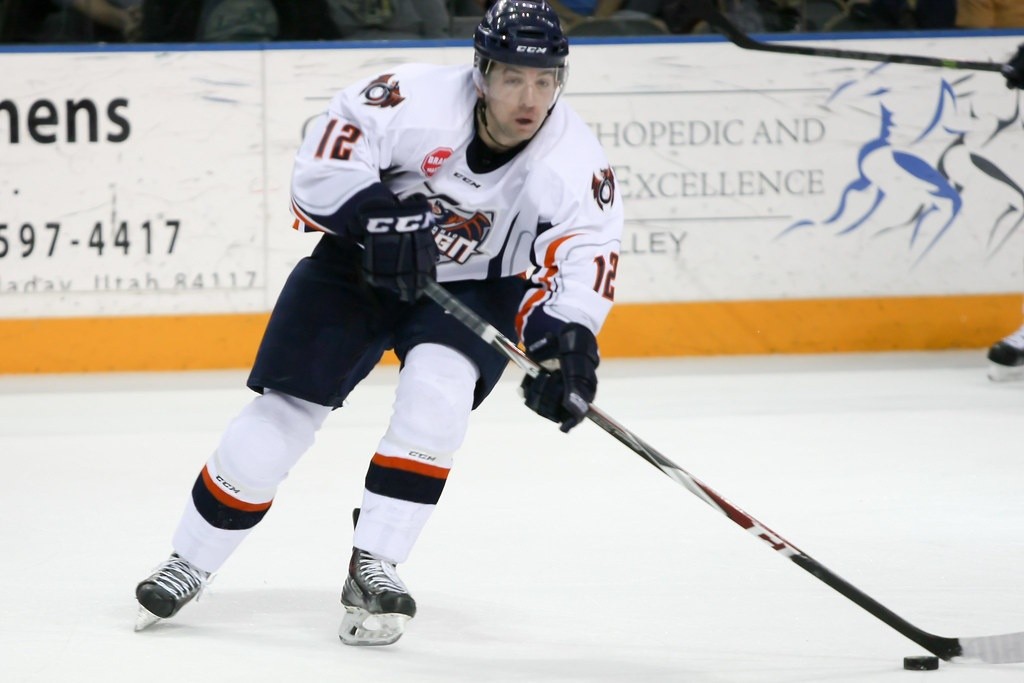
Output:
[987,44,1024,367]
[133,0,625,647]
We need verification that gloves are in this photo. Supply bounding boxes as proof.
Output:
[1004,43,1024,90]
[364,193,440,301]
[521,322,599,433]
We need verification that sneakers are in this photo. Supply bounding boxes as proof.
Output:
[133,552,211,632]
[338,508,417,647]
[986,326,1024,383]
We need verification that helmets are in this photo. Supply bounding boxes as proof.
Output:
[472,0,568,85]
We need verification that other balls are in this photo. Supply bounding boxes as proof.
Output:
[903,655,939,672]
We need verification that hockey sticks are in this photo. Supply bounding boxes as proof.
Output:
[414,277,1023,669]
